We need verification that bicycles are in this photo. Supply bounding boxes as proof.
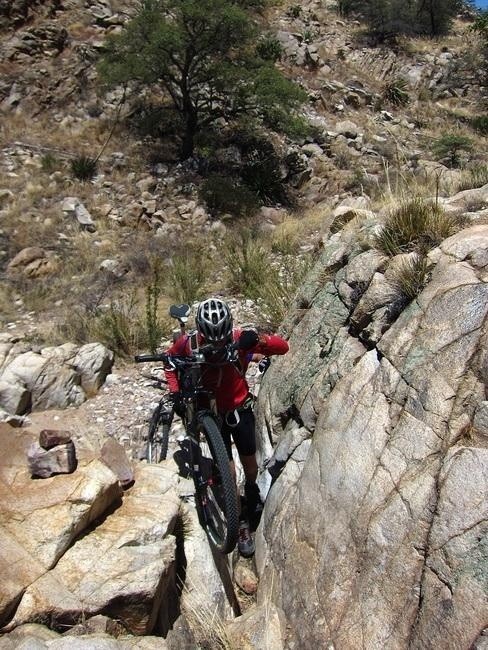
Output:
[135,305,240,554]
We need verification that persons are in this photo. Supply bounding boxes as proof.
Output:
[246,351,270,373]
[161,298,290,559]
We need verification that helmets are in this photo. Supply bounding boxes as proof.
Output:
[196,298,233,349]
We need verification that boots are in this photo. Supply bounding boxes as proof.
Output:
[244,484,263,513]
[238,520,254,556]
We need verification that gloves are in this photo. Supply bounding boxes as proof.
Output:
[239,330,259,350]
[170,391,181,405]
[258,356,270,373]
